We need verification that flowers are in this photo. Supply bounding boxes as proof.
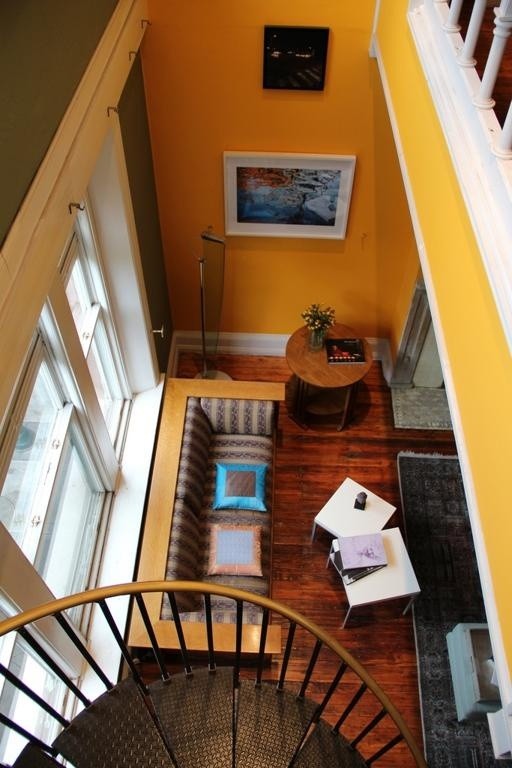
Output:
[300,303,338,330]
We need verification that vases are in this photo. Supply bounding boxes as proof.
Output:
[309,328,326,348]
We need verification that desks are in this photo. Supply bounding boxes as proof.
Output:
[285,323,373,431]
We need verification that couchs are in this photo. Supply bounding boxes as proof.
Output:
[128,379,287,668]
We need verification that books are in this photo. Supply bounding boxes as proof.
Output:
[324,338,366,364]
[332,533,389,585]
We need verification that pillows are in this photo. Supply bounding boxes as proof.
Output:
[209,524,265,578]
[213,461,269,514]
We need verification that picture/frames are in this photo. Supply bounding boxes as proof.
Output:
[223,151,357,241]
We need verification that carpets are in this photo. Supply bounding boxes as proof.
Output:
[391,388,452,430]
[396,452,511,768]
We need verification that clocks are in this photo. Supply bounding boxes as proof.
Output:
[261,24,330,91]
